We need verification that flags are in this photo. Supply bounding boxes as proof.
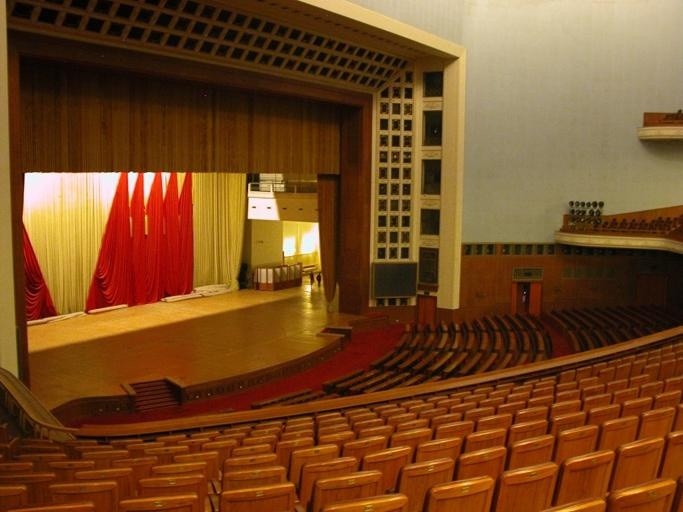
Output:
[22,222,57,321]
[145,172,164,303]
[178,171,193,296]
[163,171,179,297]
[84,171,133,312]
[128,172,145,305]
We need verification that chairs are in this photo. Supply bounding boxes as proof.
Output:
[554,108,682,253]
[1,303,682,512]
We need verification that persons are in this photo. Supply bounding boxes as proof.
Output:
[309,271,314,287]
[316,272,321,288]
